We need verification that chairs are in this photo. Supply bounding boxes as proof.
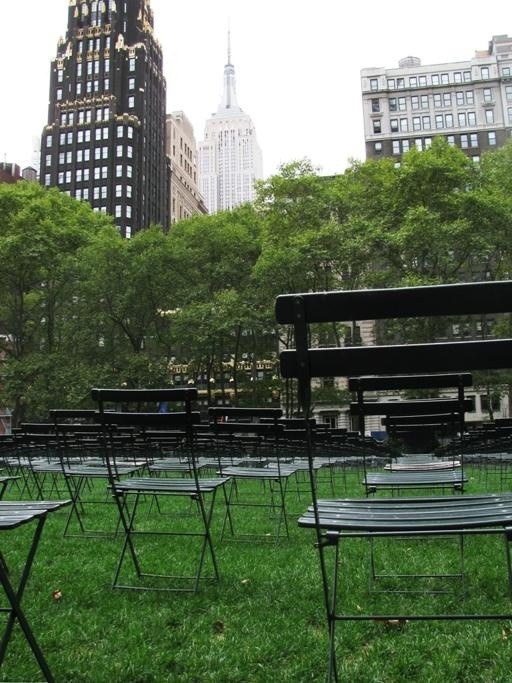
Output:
[207,404,293,550]
[348,372,475,598]
[2,367,509,502]
[88,383,232,600]
[2,500,74,682]
[274,283,509,682]
